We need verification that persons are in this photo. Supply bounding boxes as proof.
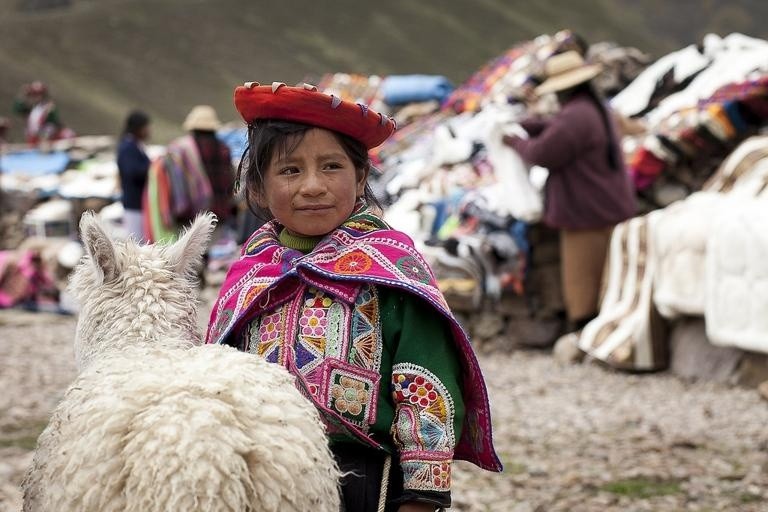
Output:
[502,50,636,331]
[203,80,502,512]
[117,112,153,213]
[142,106,234,248]
[14,81,60,145]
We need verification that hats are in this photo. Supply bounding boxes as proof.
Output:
[535,50,601,96]
[184,104,220,133]
[234,77,396,146]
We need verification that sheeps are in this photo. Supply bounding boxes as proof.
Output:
[19,209,365,512]
